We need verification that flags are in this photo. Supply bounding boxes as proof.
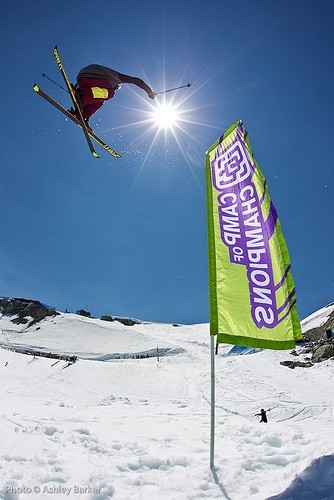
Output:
[204,120,306,350]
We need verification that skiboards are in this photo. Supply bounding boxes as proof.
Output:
[32,46,123,158]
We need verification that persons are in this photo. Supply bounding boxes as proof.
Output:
[73,64,157,123]
